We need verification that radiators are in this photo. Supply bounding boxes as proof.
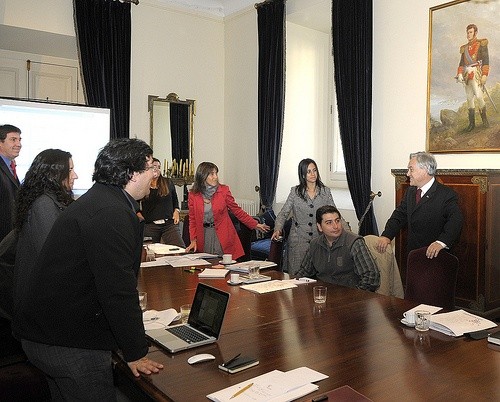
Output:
[235,199,256,216]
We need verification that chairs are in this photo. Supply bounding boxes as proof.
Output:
[404,247,460,311]
[240,217,290,270]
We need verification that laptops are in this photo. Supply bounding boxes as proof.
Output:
[144,282,231,353]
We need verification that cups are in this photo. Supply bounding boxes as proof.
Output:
[415,310,432,331]
[223,254,232,262]
[180,303,192,323]
[249,264,259,280]
[231,274,239,283]
[138,292,147,310]
[313,286,327,303]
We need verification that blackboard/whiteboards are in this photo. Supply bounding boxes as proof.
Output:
[1,97,111,195]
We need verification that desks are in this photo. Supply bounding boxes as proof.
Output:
[112,240,500,402]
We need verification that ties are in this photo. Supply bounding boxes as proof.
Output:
[415,188,422,205]
[10,161,17,179]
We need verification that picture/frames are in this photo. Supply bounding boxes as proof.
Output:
[426,0,500,155]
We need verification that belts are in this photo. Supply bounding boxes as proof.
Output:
[203,223,215,227]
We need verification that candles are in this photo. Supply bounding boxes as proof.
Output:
[163,158,183,178]
[176,158,192,177]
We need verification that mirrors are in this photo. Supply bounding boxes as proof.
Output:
[147,94,197,186]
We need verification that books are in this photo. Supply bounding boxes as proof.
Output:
[488,330,500,345]
[430,309,497,337]
[218,356,259,374]
[199,267,230,278]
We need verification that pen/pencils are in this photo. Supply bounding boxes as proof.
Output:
[169,248,179,251]
[142,317,159,321]
[183,268,195,274]
[191,267,203,272]
[229,382,254,400]
[222,352,242,367]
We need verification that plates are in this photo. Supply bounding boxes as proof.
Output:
[219,260,237,264]
[227,279,243,285]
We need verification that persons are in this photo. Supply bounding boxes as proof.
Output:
[20,137,164,400]
[135,158,187,249]
[270,158,336,276]
[293,205,381,292]
[0,125,76,329]
[185,161,271,261]
[376,151,463,260]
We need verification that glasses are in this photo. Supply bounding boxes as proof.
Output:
[144,164,157,170]
